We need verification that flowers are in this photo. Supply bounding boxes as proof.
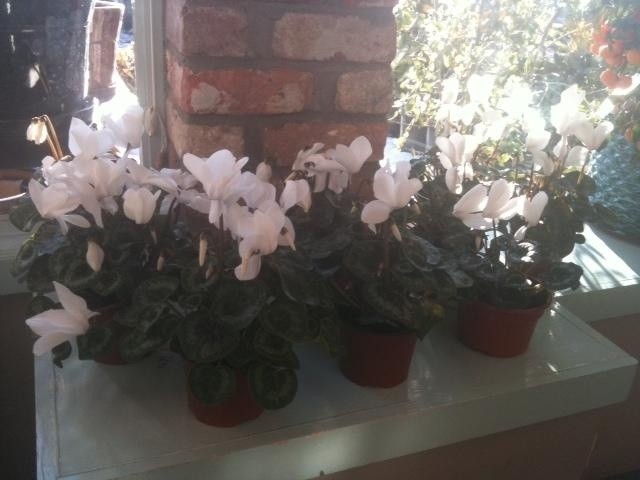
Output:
[280,136,472,341]
[167,150,315,408]
[14,63,167,368]
[437,76,611,312]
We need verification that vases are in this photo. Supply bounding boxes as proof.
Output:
[87,310,130,364]
[466,296,542,357]
[341,322,415,386]
[187,359,265,427]
